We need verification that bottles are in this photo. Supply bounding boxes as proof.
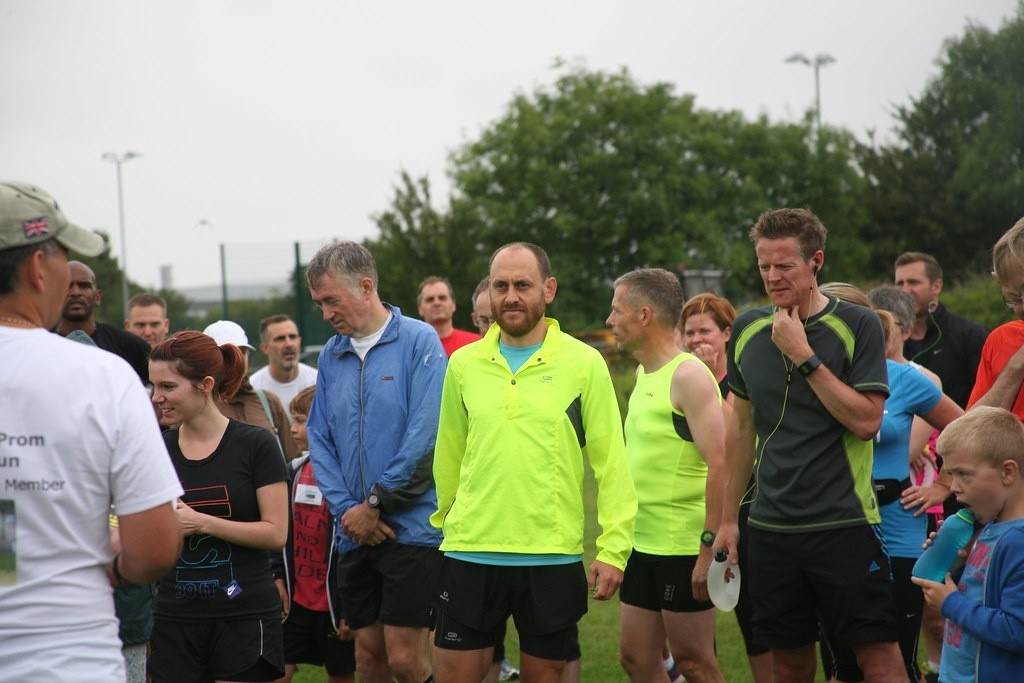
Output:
[707,548,740,612]
[912,508,974,583]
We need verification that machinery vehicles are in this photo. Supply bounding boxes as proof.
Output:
[572,264,725,366]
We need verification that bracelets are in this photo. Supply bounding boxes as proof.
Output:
[700,529,715,545]
[112,554,131,596]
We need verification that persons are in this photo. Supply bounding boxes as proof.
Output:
[414,277,529,683]
[304,241,448,682]
[964,216,1024,426]
[0,177,189,683]
[821,278,944,683]
[142,312,355,683]
[428,238,636,682]
[603,268,731,683]
[658,292,781,683]
[908,409,1024,683]
[893,252,991,470]
[709,204,912,683]
[50,262,171,683]
[867,281,945,537]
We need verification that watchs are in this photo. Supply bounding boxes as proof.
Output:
[796,353,823,378]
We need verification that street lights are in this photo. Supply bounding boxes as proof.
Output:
[102,148,138,321]
[782,53,836,139]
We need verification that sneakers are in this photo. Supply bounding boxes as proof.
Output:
[498,659,520,682]
[666,662,685,683]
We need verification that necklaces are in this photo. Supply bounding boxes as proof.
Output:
[0,314,37,327]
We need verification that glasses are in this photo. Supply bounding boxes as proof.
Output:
[999,284,1024,308]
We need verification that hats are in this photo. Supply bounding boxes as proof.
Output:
[202,320,256,353]
[0,178,106,257]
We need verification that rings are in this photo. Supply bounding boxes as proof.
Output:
[920,497,923,501]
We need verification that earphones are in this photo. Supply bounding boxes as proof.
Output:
[813,258,819,275]
